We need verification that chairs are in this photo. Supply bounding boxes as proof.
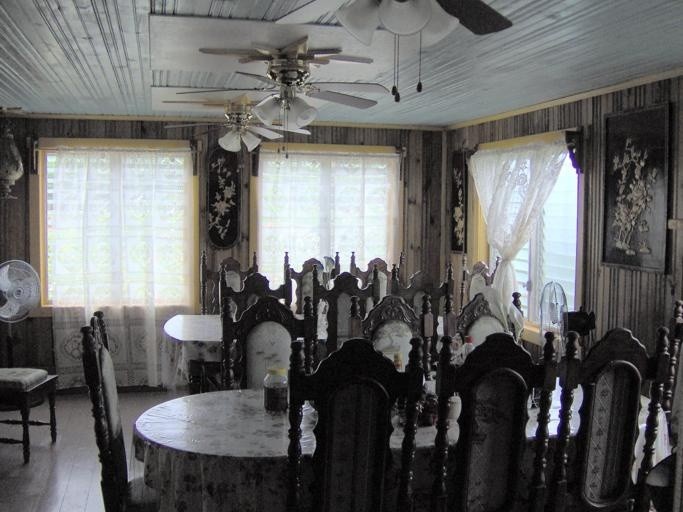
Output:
[280,334,424,510]
[199,251,258,315]
[430,331,556,511]
[460,256,502,307]
[647,300,682,511]
[312,264,380,350]
[351,251,407,301]
[284,251,340,314]
[220,296,314,389]
[391,262,455,321]
[552,326,671,512]
[217,269,293,383]
[82,312,157,510]
[349,294,435,382]
[444,290,525,347]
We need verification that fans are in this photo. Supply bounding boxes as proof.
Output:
[0,260,45,411]
[176,36,390,109]
[162,93,312,140]
[275,0,513,34]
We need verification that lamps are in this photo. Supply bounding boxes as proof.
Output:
[334,0,459,101]
[252,86,319,158]
[0,107,24,200]
[218,124,261,152]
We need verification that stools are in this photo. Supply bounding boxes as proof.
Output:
[0,367,58,466]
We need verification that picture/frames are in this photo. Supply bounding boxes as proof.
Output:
[206,147,242,250]
[450,148,468,251]
[600,103,670,273]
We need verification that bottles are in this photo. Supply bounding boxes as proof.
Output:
[463,335,475,357]
[263,368,289,412]
[391,352,406,371]
